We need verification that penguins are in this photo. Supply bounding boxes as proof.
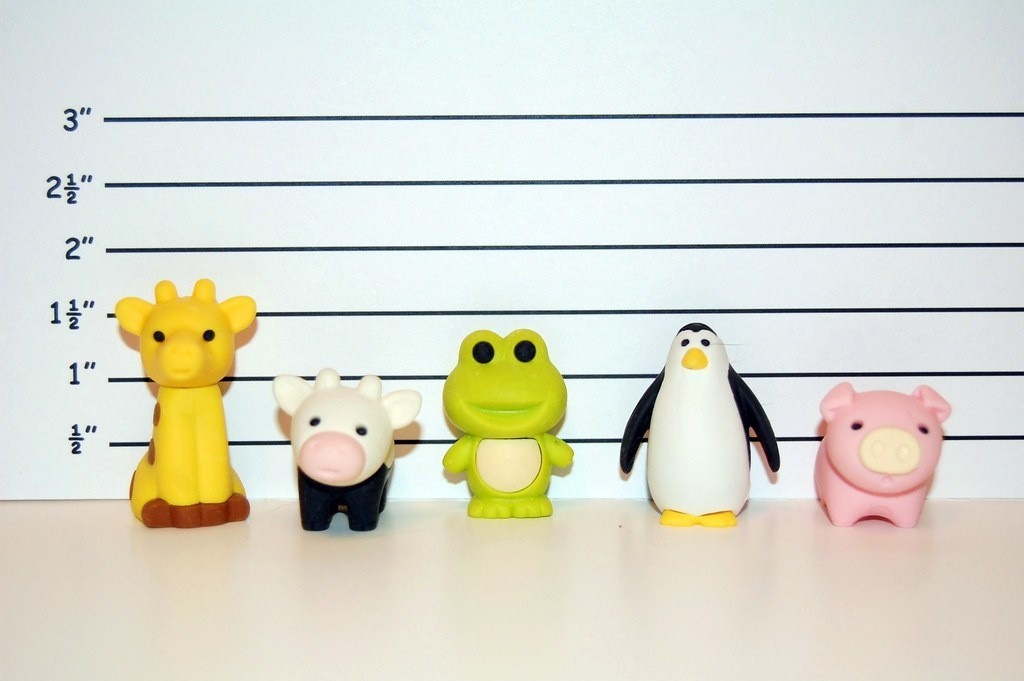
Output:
[620,323,780,531]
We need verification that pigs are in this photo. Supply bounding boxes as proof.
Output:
[815,382,951,527]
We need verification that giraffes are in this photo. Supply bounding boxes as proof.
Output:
[115,278,257,529]
